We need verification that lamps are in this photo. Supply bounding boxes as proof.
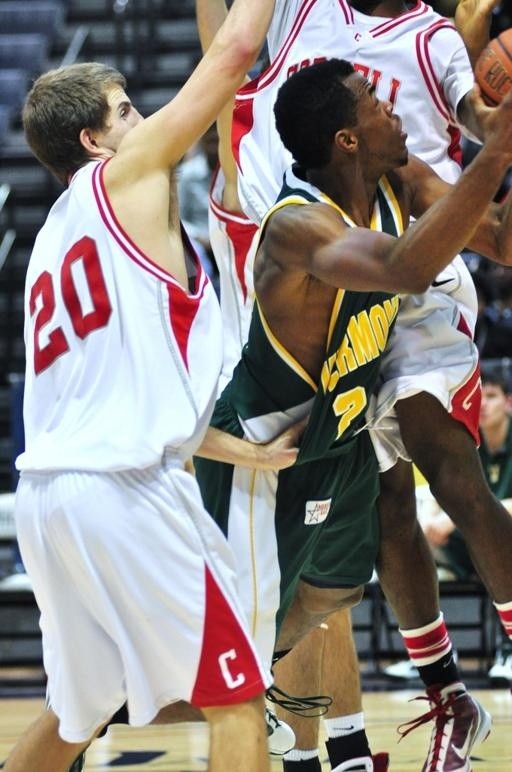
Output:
[0,0,76,151]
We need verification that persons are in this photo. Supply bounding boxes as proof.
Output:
[451,0,496,64]
[232,1,511,771]
[0,1,277,770]
[383,359,510,687]
[191,1,391,772]
[70,60,510,772]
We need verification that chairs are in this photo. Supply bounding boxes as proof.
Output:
[385,660,419,678]
[487,647,512,679]
[422,680,494,772]
[328,752,389,771]
[267,707,296,757]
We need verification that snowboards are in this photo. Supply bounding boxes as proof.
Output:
[473,29,512,106]
